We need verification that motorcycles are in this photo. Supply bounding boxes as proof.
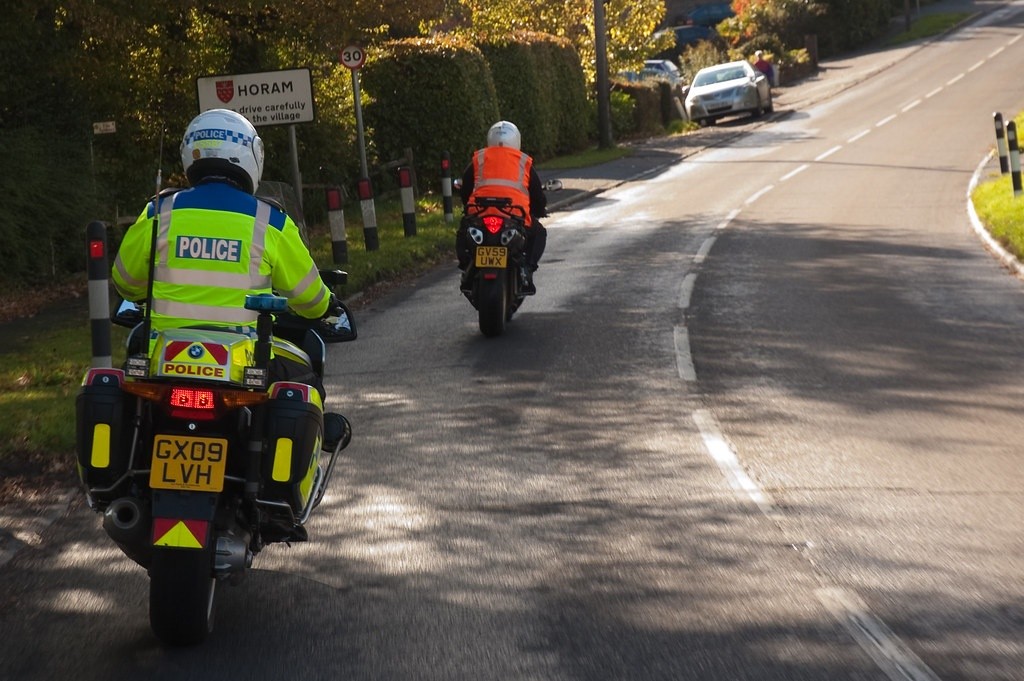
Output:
[451,176,563,337]
[71,268,360,649]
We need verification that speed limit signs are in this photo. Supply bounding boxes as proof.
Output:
[338,43,365,69]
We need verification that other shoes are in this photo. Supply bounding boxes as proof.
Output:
[518,268,536,296]
[460,270,471,290]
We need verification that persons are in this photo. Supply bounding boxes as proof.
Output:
[110,109,340,543]
[456,121,547,296]
[753,49,774,81]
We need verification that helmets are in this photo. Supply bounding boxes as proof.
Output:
[487,121,522,151]
[180,108,266,196]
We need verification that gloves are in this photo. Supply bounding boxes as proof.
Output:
[324,292,338,317]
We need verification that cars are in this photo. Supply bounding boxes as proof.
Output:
[676,2,736,30]
[616,59,686,92]
[679,59,773,127]
[641,25,730,72]
